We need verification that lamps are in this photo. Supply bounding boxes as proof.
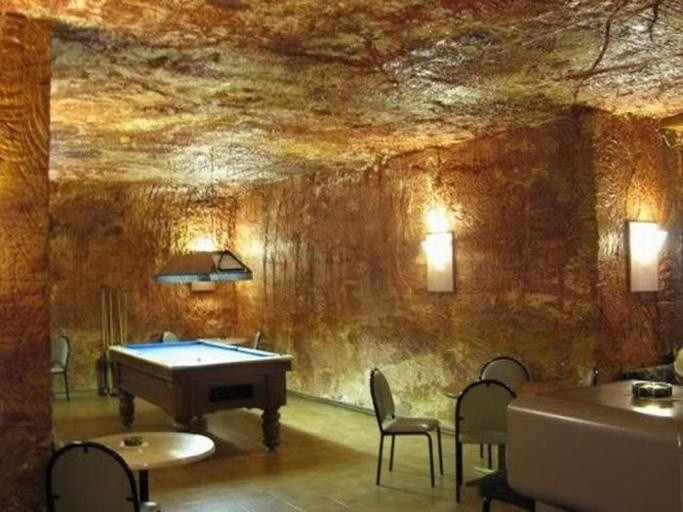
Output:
[191,239,215,291]
[422,233,454,291]
[626,219,668,293]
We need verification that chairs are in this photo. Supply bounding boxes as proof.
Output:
[47,441,158,512]
[479,357,530,469]
[455,379,517,502]
[370,368,443,488]
[49,335,70,402]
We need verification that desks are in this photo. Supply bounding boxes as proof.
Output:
[107,338,293,452]
[442,380,520,398]
[82,432,215,512]
